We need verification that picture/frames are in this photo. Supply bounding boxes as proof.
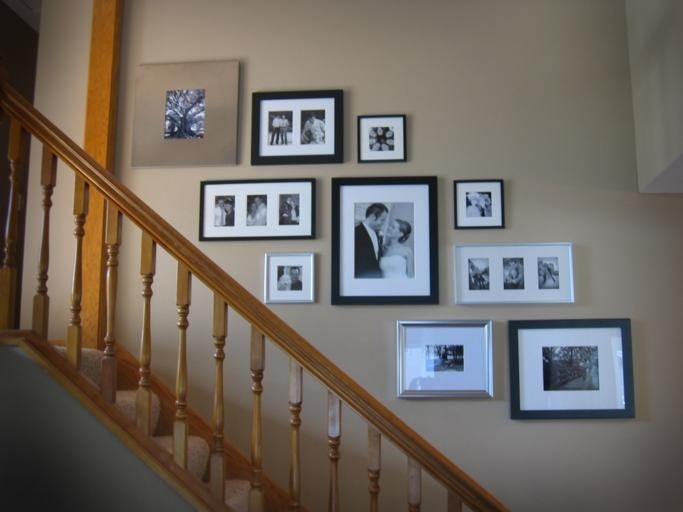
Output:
[251,90,343,164]
[330,176,439,304]
[264,253,315,305]
[395,320,493,399]
[508,319,634,419]
[453,179,504,228]
[453,243,574,304]
[358,115,406,161]
[200,181,314,238]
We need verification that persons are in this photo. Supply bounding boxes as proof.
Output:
[270,113,281,145]
[380,218,413,279]
[247,196,267,226]
[290,267,302,290]
[280,114,289,145]
[223,200,235,226]
[281,197,299,224]
[215,198,227,226]
[302,113,325,145]
[355,202,389,278]
